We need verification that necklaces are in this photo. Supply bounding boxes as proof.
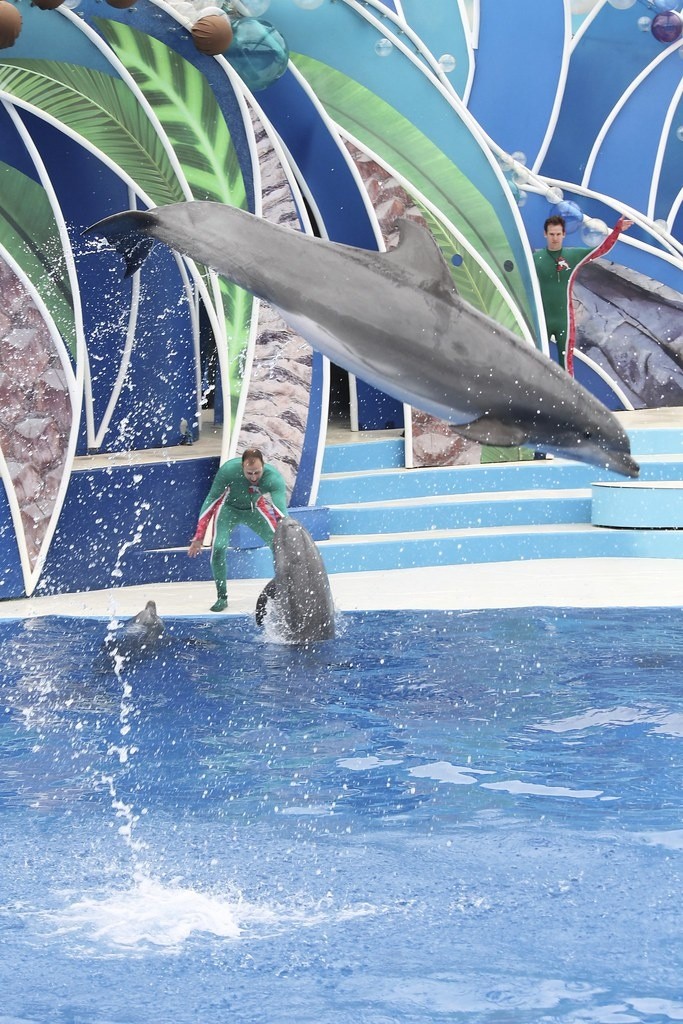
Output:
[248,491,258,513]
[547,249,564,283]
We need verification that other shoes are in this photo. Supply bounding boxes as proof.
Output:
[210,599,227,612]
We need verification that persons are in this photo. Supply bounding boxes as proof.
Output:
[187,448,290,612]
[532,213,635,379]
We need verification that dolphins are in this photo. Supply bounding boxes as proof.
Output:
[91,601,195,679]
[79,197,644,483]
[256,514,337,645]
